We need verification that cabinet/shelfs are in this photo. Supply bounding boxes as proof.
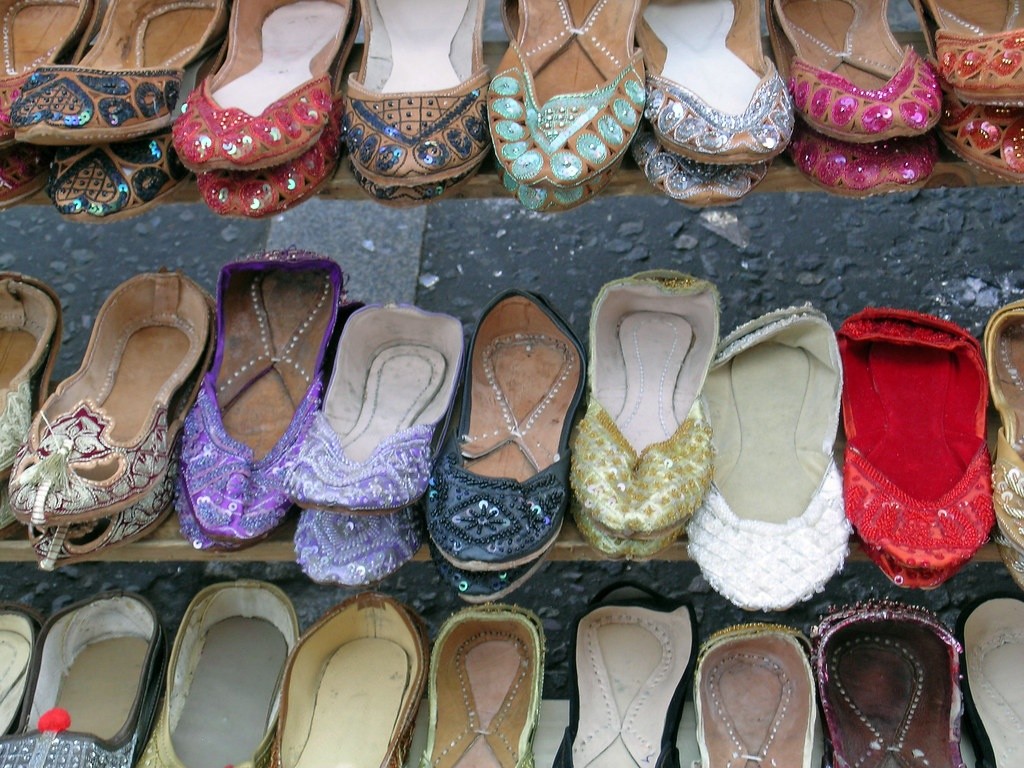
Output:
[0,33,1024,768]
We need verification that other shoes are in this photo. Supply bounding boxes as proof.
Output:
[0,0,1024,768]
